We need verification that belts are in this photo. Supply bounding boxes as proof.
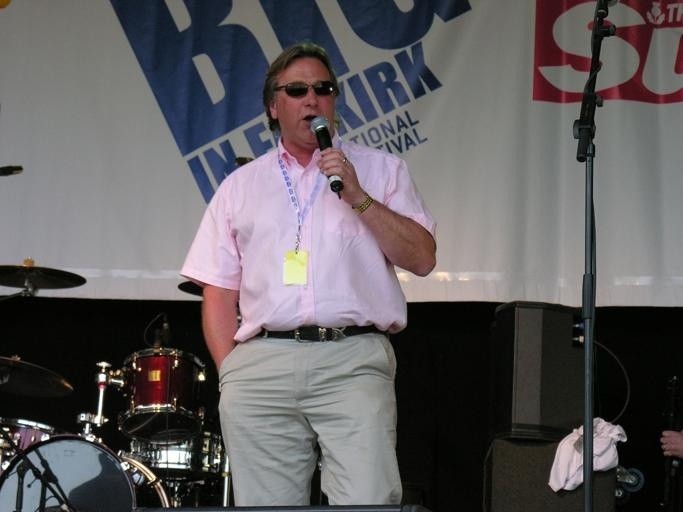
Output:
[257,324,383,343]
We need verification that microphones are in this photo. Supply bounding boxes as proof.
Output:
[309,114,344,196]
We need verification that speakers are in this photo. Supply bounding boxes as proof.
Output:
[490,430,616,512]
[510,302,598,442]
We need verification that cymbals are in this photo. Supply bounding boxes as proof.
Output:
[178,280,203,296]
[0,265,87,289]
[0,357,74,397]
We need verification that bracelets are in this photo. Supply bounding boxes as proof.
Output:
[352,193,374,215]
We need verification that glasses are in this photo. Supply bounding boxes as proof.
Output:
[275,79,335,99]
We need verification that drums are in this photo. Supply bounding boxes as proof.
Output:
[0,434,170,512]
[117,347,208,446]
[150,428,223,483]
[1,417,54,478]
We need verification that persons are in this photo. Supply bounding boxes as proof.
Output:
[180,44,437,512]
[660,430,683,458]
